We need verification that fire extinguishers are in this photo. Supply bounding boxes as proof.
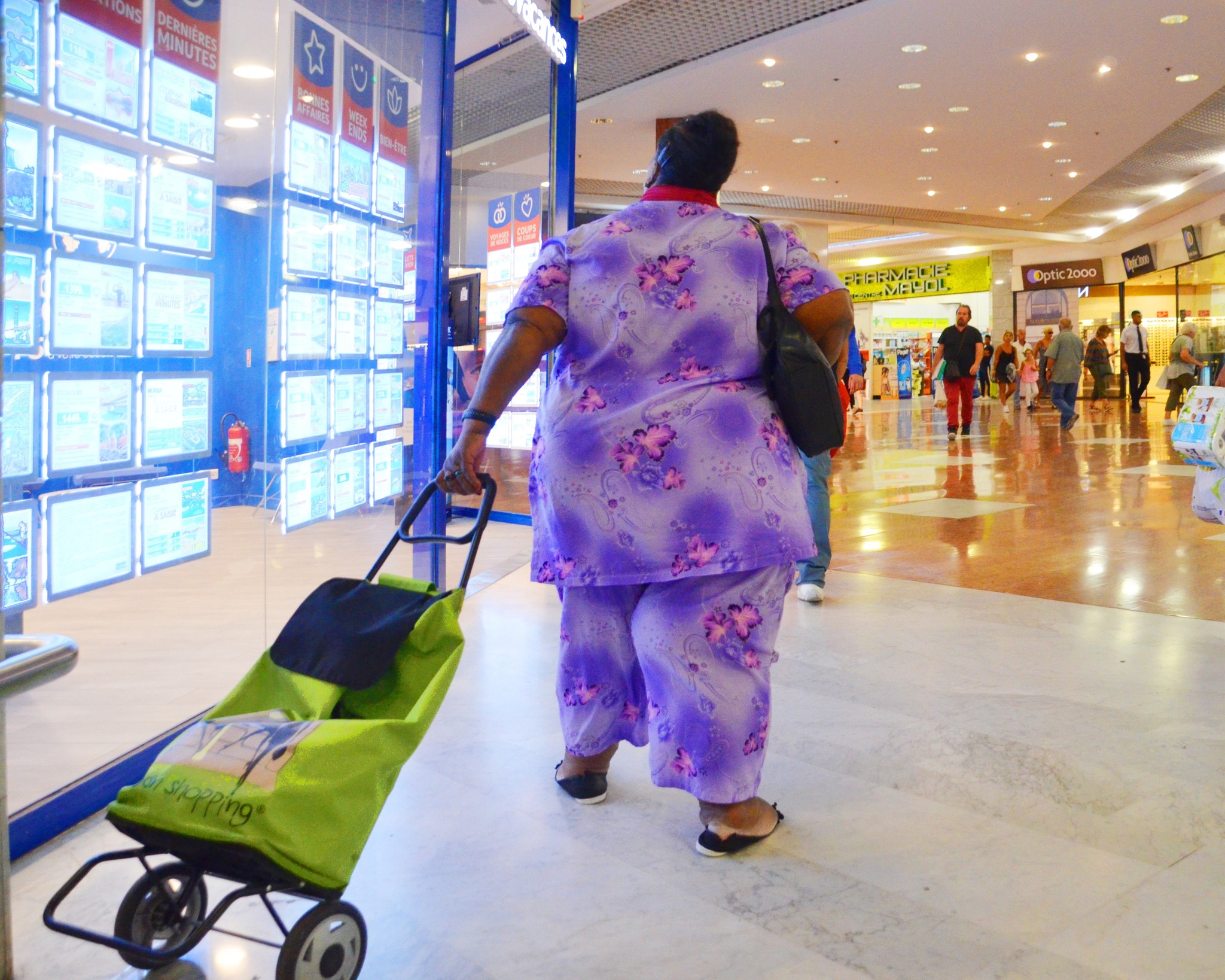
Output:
[215,413,249,473]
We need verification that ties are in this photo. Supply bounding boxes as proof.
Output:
[1136,326,1144,352]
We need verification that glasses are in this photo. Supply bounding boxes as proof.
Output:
[1046,331,1053,334]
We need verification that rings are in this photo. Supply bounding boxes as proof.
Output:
[443,474,455,482]
[454,469,462,477]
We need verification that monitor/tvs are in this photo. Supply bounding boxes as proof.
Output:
[448,272,482,348]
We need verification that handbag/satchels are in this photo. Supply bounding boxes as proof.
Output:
[747,215,845,459]
[1006,362,1017,382]
[933,380,948,411]
[1156,365,1172,390]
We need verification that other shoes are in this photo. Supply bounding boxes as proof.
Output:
[696,802,784,856]
[1132,404,1142,413]
[1027,406,1031,411]
[847,406,863,414]
[555,760,608,804]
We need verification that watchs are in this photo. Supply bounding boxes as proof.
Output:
[1045,368,1051,371]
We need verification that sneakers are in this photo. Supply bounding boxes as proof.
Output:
[1162,418,1176,426]
[981,395,991,400]
[1003,405,1010,413]
[960,422,971,437]
[1065,414,1079,431]
[947,425,958,440]
[974,395,984,400]
[797,583,825,601]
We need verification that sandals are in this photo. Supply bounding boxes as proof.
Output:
[1102,404,1113,412]
[1089,404,1100,411]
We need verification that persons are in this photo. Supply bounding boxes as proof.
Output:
[785,324,867,604]
[981,335,993,398]
[1120,310,1153,413]
[881,367,891,395]
[1044,317,1085,431]
[1083,325,1119,413]
[1162,322,1204,426]
[435,109,854,857]
[991,326,1054,413]
[454,351,486,408]
[932,304,984,440]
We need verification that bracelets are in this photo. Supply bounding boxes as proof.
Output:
[461,409,496,429]
